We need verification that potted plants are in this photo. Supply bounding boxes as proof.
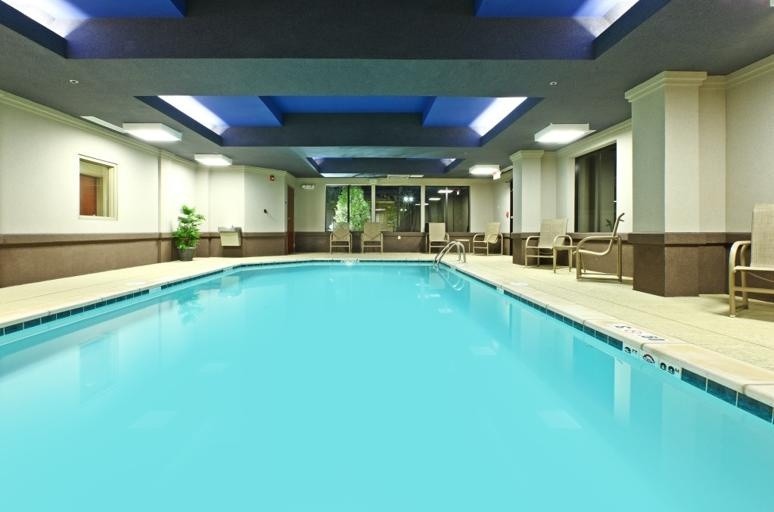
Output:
[172,204,208,261]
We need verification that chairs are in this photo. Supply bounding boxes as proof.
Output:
[523,217,577,275]
[573,210,627,283]
[726,196,774,320]
[425,222,451,253]
[329,221,353,254]
[360,221,384,253]
[472,221,504,257]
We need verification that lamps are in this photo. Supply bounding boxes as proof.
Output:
[533,122,594,146]
[468,163,501,176]
[193,153,234,168]
[121,121,185,145]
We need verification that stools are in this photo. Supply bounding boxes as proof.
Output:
[456,239,472,255]
